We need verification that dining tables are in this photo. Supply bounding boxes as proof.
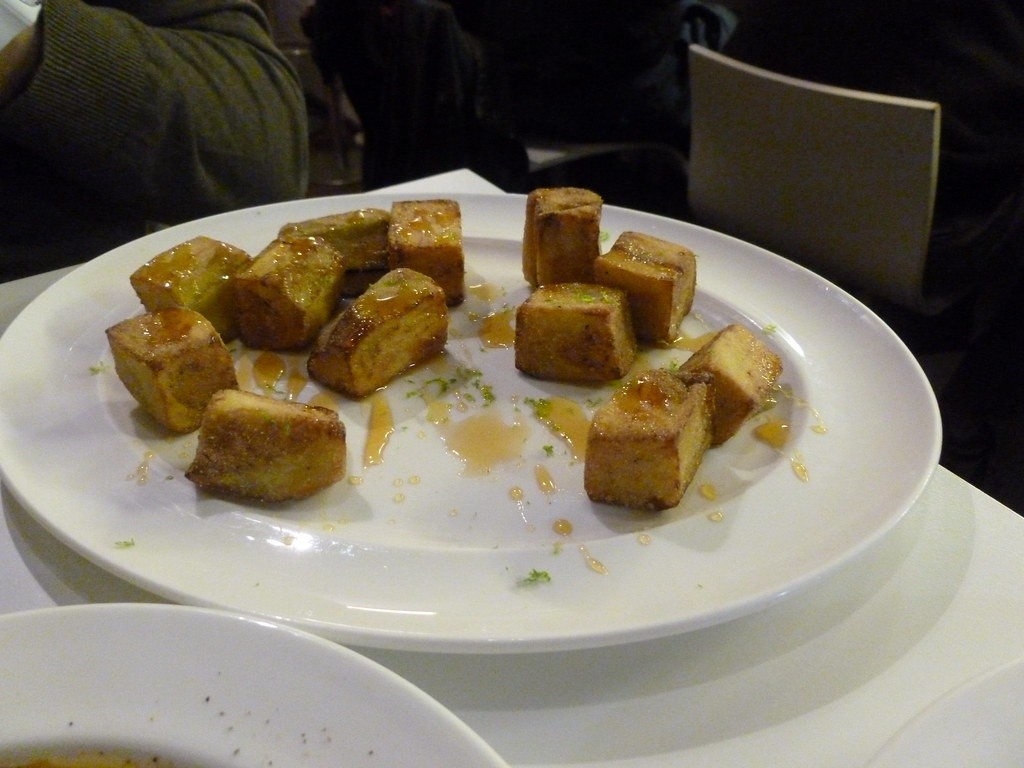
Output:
[0,165,1024,767]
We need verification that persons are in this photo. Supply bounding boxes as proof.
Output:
[0,0,309,288]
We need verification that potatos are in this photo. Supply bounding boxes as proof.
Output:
[104,186,784,514]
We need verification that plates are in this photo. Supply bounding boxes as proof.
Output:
[0,194,943,654]
[866,657,1024,768]
[0,603,510,768]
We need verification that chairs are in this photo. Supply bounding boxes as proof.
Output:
[522,42,1024,418]
